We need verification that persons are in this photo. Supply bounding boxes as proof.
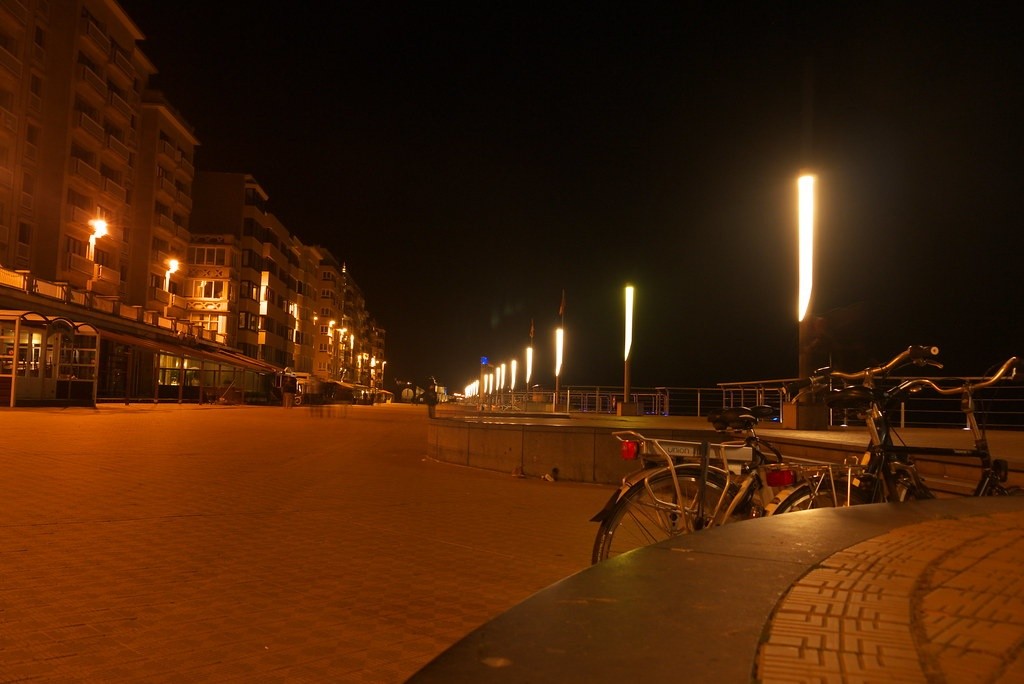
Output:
[369,391,375,406]
[438,398,439,403]
[363,391,368,405]
[411,396,414,406]
[283,376,297,408]
[424,383,439,419]
[377,393,382,406]
[415,396,419,406]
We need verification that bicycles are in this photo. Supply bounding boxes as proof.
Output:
[593,345,1024,564]
